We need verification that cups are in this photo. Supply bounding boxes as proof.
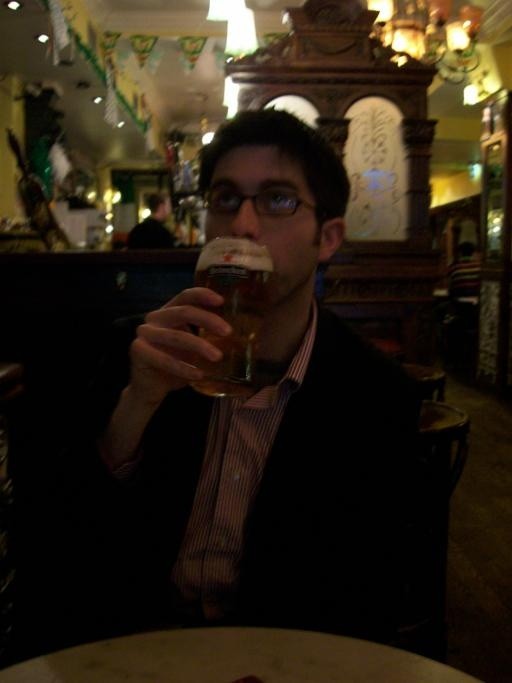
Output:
[184,237,277,399]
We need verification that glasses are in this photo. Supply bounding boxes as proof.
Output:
[201,189,320,217]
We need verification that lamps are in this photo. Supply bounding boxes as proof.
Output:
[367,0,486,90]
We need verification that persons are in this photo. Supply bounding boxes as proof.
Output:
[447,241,495,347]
[8,108,424,636]
[126,217,178,249]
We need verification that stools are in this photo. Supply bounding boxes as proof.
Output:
[420,399,469,496]
[400,358,450,402]
